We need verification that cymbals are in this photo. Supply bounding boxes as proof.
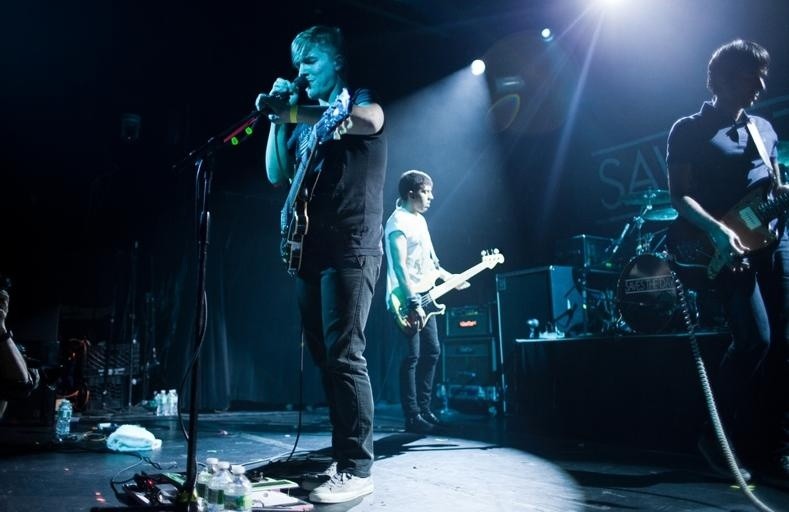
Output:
[644,208,679,221]
[624,190,672,206]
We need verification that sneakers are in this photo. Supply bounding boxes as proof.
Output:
[692,421,755,488]
[754,451,789,494]
[308,469,376,505]
[301,462,338,492]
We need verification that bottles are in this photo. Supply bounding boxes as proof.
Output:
[155,389,180,418]
[195,456,253,511]
[56,398,72,436]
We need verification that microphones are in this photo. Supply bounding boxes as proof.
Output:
[278,77,309,101]
[456,371,476,378]
[567,303,577,326]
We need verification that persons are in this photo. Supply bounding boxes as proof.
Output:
[381,169,473,435]
[253,23,388,507]
[0,289,31,387]
[664,36,788,486]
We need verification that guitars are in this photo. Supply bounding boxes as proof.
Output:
[664,177,789,291]
[281,88,352,273]
[389,248,505,339]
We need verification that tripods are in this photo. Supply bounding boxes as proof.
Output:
[90,102,315,512]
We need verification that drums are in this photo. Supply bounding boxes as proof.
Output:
[617,253,677,334]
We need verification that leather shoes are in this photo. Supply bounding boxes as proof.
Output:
[421,407,449,427]
[406,414,447,434]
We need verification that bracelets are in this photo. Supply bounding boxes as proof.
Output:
[0,329,13,341]
[289,104,299,124]
